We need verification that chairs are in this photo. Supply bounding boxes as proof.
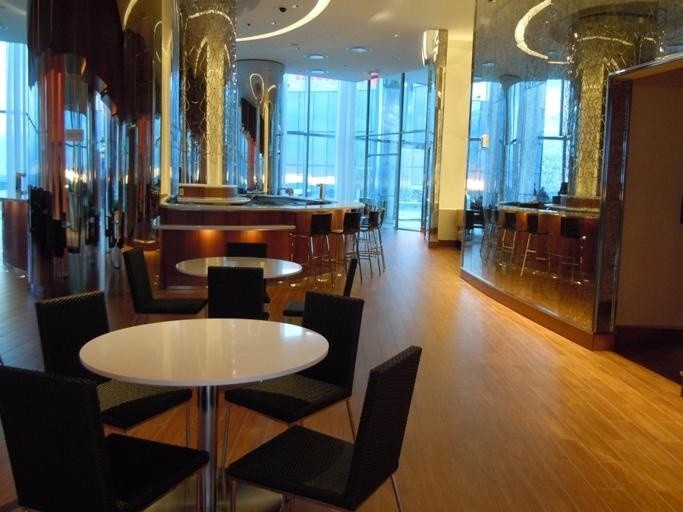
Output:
[464,204,586,287]
[288,202,386,290]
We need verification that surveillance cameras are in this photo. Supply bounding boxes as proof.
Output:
[279,7,287,13]
